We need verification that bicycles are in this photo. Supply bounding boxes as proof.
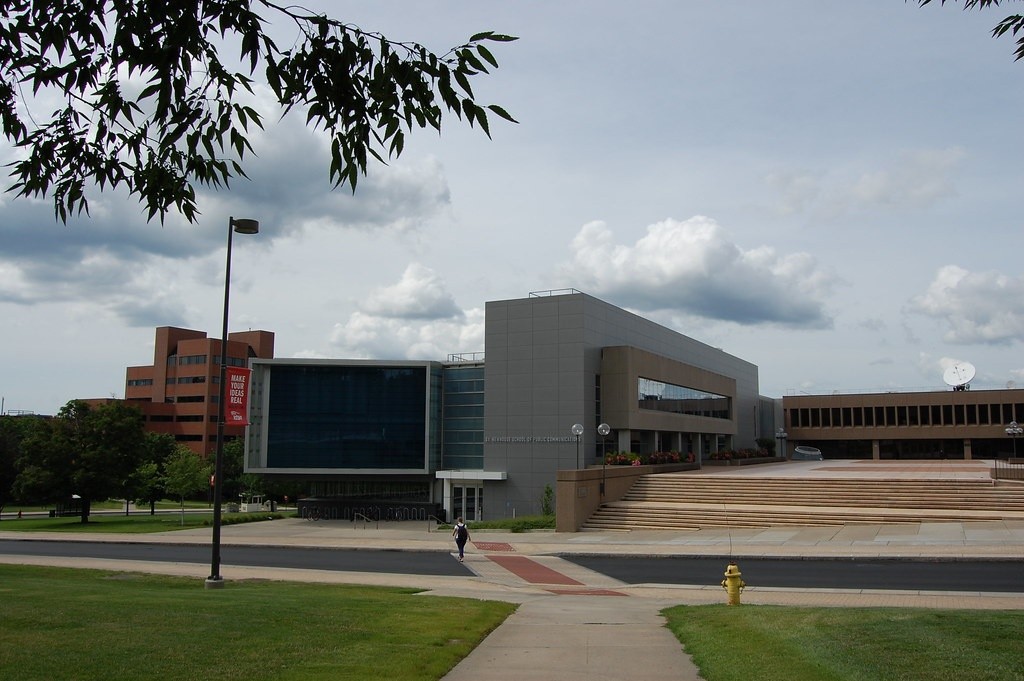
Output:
[304,505,405,522]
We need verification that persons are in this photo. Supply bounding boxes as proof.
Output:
[453,517,471,563]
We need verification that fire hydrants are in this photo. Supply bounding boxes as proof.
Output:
[721,561,746,605]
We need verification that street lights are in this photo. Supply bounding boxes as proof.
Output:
[1005,421,1023,458]
[598,422,610,497]
[776,428,789,456]
[207,217,261,580]
[572,423,584,470]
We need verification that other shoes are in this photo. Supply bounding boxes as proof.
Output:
[458,556,464,562]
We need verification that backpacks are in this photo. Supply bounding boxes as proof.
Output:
[456,524,468,540]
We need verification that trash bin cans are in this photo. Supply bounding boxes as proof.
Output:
[436,508,446,524]
